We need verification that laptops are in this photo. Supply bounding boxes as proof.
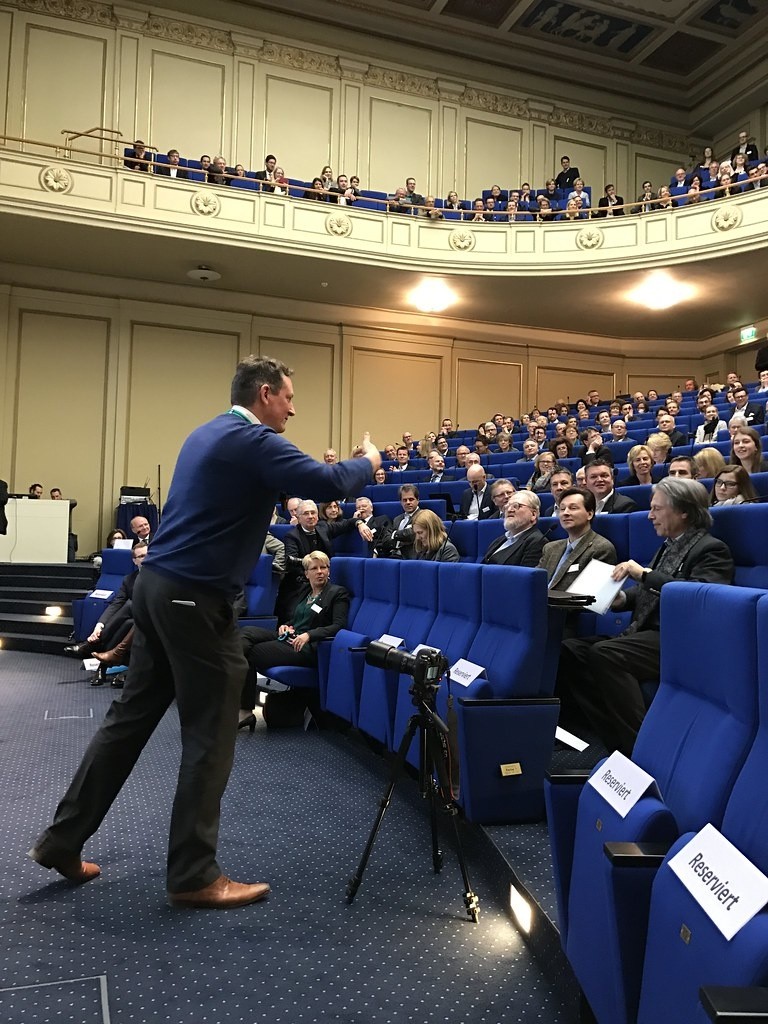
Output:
[430,493,456,514]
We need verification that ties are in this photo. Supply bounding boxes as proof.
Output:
[431,475,439,483]
[142,539,147,545]
[398,515,410,531]
[548,544,573,588]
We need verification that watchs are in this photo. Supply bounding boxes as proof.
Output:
[357,520,364,527]
[642,568,652,583]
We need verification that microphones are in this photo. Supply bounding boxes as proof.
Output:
[709,416,715,442]
[369,424,503,562]
[650,409,654,416]
[691,430,697,456]
[520,523,559,566]
[662,448,672,478]
[611,467,618,514]
[619,390,621,395]
[567,396,569,404]
[677,385,680,392]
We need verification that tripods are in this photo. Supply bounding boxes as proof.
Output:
[344,686,480,924]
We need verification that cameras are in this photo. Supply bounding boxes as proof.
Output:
[365,641,449,686]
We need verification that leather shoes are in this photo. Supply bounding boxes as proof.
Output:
[91,650,122,666]
[90,664,107,686]
[168,875,271,907]
[112,672,128,687]
[64,642,91,659]
[27,842,101,881]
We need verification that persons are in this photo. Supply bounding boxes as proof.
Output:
[29,356,381,906]
[51,488,62,500]
[412,509,460,563]
[29,484,43,499]
[386,155,591,221]
[595,184,625,218]
[259,495,391,628]
[0,480,9,535]
[384,484,422,560]
[482,490,550,569]
[533,487,616,641]
[323,332,768,520]
[237,551,348,729]
[630,131,768,215]
[559,477,733,758]
[304,166,361,206]
[64,517,152,686]
[124,140,290,196]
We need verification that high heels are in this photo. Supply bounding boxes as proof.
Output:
[238,714,257,731]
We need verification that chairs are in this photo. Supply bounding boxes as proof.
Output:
[542,580,768,1023]
[326,557,402,728]
[429,563,560,823]
[668,158,767,206]
[259,557,367,689]
[75,382,768,639]
[394,562,484,787]
[635,595,768,1023]
[358,560,443,751]
[123,147,591,222]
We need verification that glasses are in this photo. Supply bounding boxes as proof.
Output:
[134,554,146,559]
[494,492,512,498]
[714,478,738,489]
[502,502,533,512]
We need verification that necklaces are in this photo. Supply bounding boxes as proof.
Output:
[307,593,320,601]
